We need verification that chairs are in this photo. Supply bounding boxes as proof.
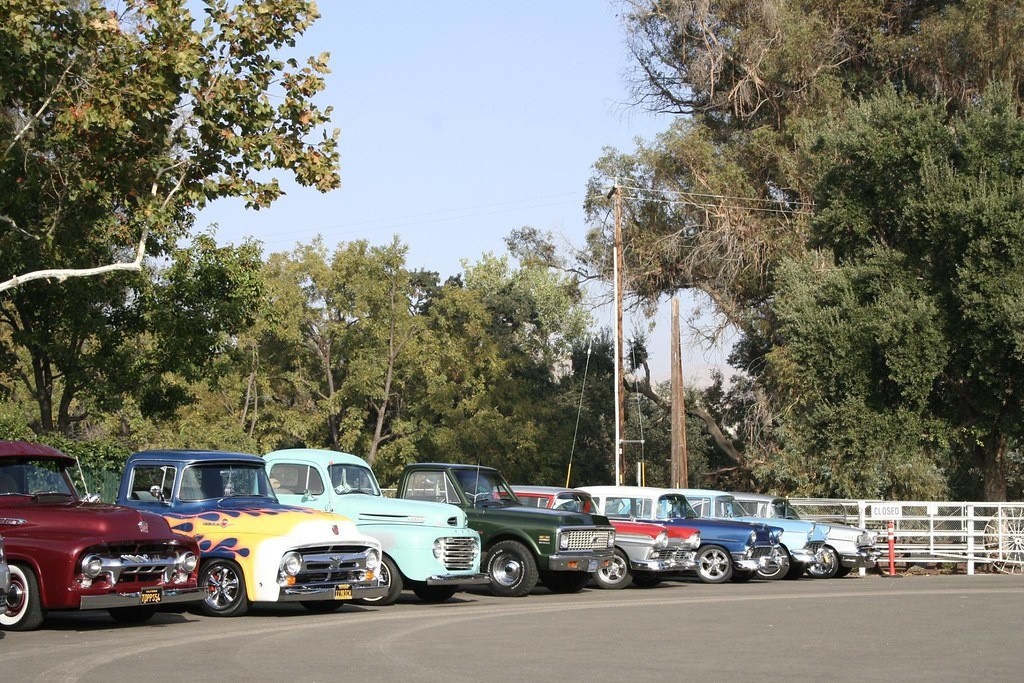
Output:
[0,473,17,493]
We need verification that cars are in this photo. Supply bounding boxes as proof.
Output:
[394,462,614,599]
[492,484,702,590]
[114,447,389,618]
[0,439,210,632]
[549,485,884,583]
[253,448,482,609]
